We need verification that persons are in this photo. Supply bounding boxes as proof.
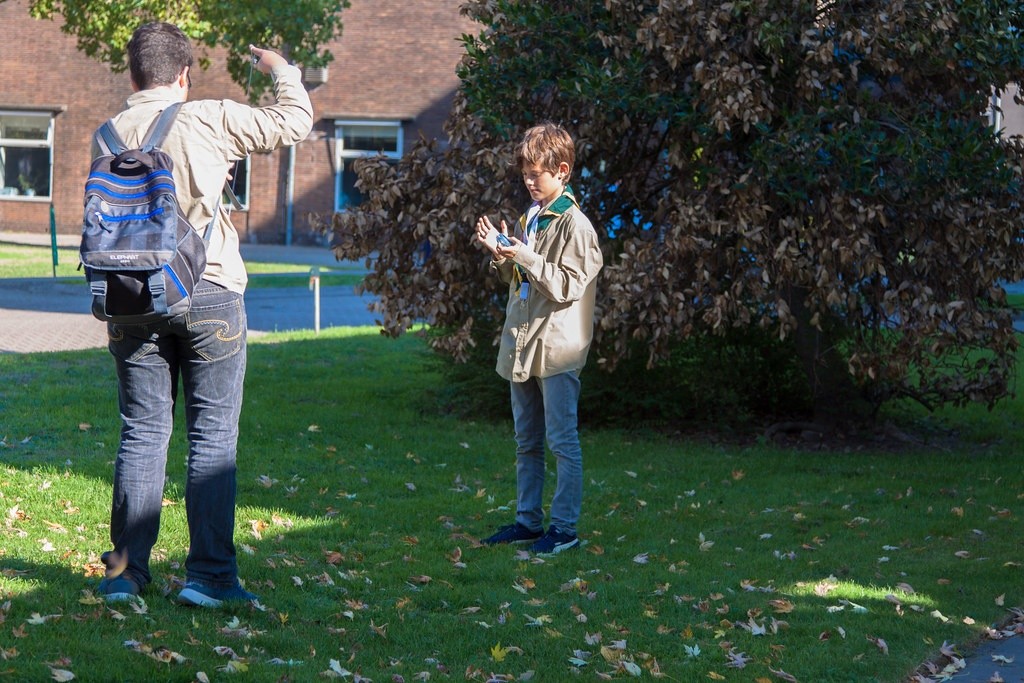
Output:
[92,23,314,606]
[476,125,603,558]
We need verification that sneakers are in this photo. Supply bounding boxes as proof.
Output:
[526,524,579,552]
[91,571,141,602]
[479,522,545,547]
[177,580,259,607]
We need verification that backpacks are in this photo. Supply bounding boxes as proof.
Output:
[76,104,244,326]
